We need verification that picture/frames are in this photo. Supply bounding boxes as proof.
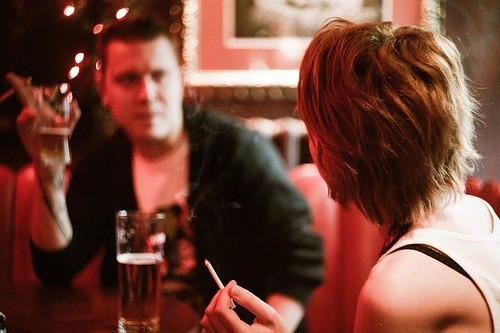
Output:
[184,0,440,86]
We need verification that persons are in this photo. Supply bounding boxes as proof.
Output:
[202,15,500,333]
[5,17,328,332]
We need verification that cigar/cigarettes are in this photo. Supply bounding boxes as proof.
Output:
[0,78,32,101]
[204,260,236,309]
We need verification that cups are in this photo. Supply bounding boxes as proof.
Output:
[34,83,73,168]
[115,210,167,333]
